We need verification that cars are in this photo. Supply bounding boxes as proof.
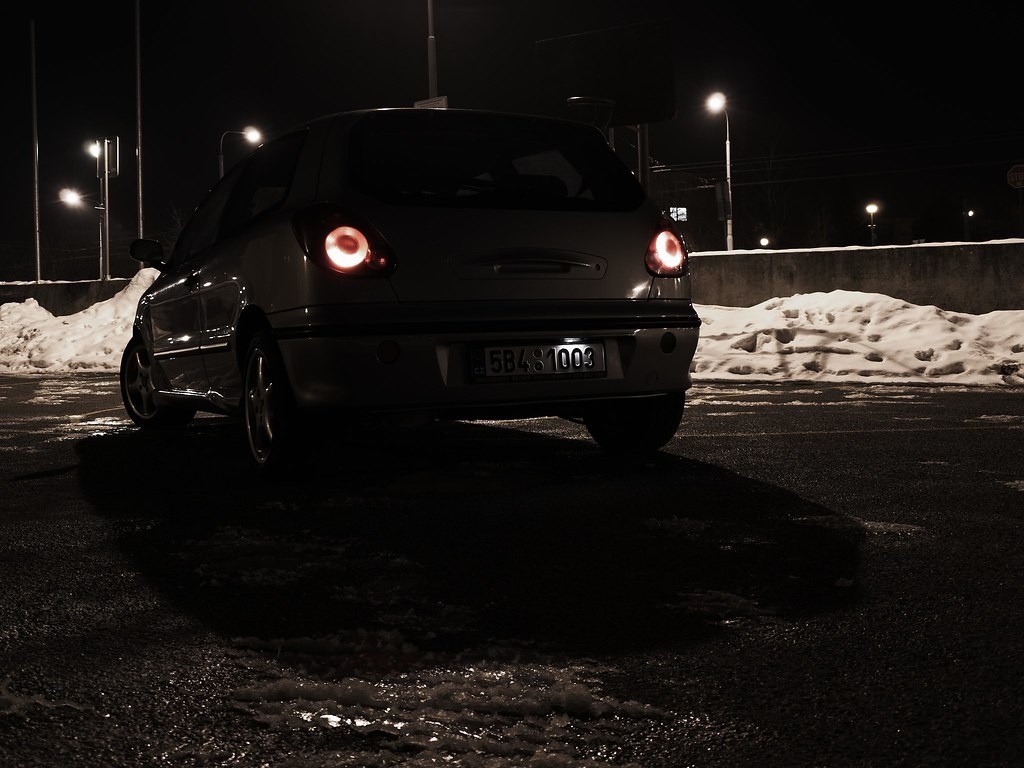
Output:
[119,108,702,482]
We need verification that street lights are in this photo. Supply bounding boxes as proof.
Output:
[963,208,974,241]
[59,140,106,280]
[217,124,265,181]
[865,202,880,246]
[705,91,734,250]
